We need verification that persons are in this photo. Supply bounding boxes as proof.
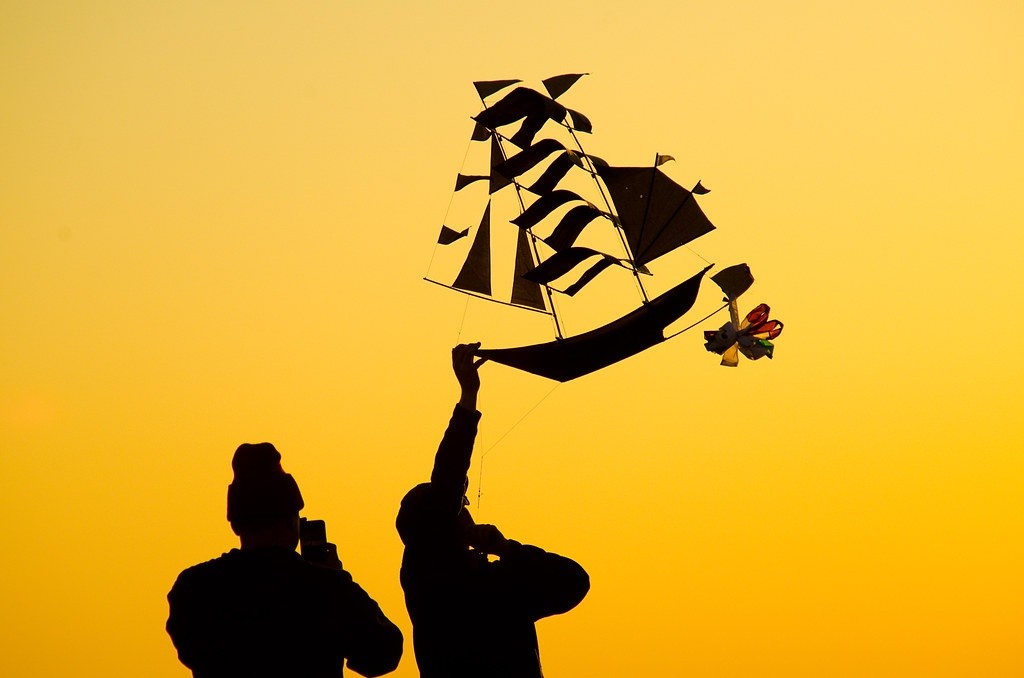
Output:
[166,444,404,678]
[396,342,590,678]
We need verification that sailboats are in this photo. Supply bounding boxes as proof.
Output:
[422,72,755,386]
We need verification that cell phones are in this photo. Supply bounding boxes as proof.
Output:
[299,520,327,568]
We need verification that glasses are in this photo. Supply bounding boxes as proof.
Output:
[463,496,470,506]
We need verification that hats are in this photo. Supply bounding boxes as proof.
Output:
[225,442,304,522]
